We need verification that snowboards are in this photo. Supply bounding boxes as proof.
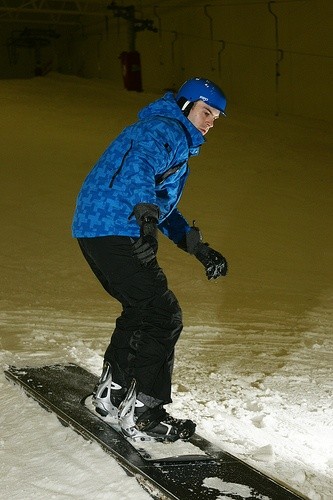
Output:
[4,362,310,500]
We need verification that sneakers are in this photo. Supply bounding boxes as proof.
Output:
[119,379,195,438]
[92,362,128,416]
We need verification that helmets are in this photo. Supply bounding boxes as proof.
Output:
[177,78,227,117]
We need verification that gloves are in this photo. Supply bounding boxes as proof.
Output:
[133,203,158,264]
[177,227,227,279]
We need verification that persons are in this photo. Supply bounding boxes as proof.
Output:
[73,79,227,445]
